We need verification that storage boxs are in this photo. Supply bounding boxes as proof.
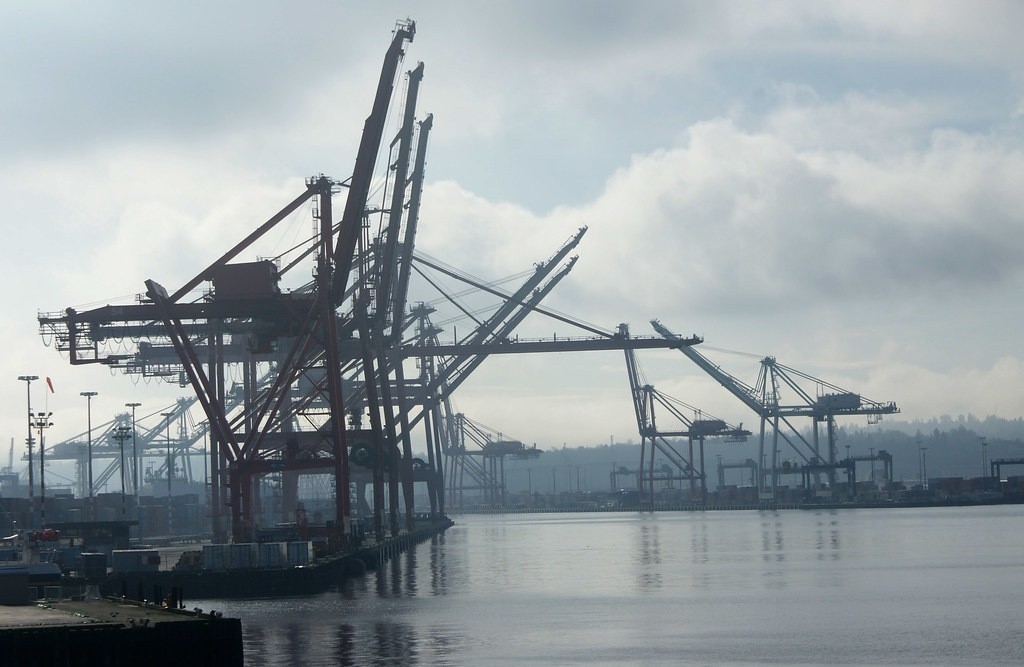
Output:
[0,492,376,569]
[717,475,1024,503]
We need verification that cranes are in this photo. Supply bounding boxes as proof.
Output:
[36,15,705,538]
[615,318,897,496]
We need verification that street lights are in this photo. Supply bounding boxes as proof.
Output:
[777,450,784,485]
[979,436,988,477]
[149,461,156,475]
[200,420,212,522]
[161,412,177,535]
[917,441,924,484]
[717,455,724,482]
[869,448,876,481]
[126,402,143,505]
[921,448,928,490]
[113,426,131,514]
[28,410,53,525]
[17,376,42,529]
[844,445,851,459]
[78,392,99,510]
[527,464,593,497]
[658,459,664,487]
[763,454,769,488]
